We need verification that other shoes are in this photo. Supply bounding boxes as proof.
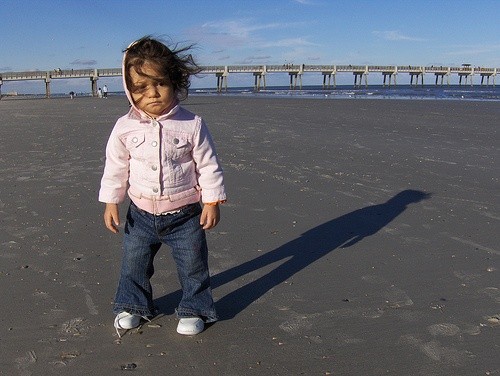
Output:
[176,316,207,336]
[114,312,144,329]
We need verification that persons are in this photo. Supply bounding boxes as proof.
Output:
[103,84,108,100]
[98,37,226,335]
[98,87,102,99]
